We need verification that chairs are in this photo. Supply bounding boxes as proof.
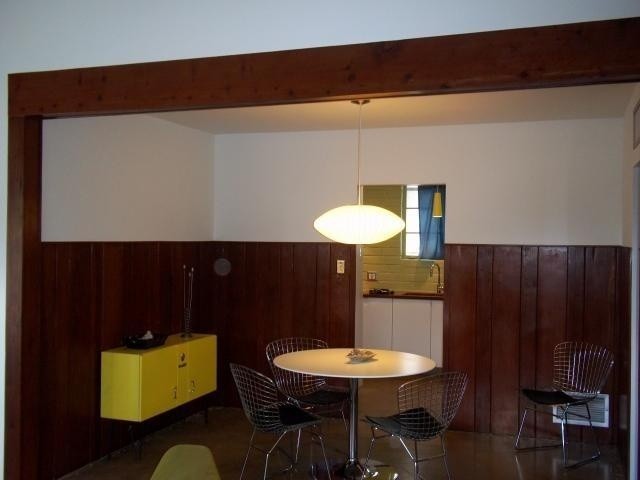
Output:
[515,341,615,470]
[228,363,332,480]
[362,372,472,480]
[265,337,352,464]
[149,444,221,480]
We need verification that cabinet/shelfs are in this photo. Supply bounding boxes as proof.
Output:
[100,332,218,463]
[364,297,443,421]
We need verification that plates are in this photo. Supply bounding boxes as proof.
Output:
[122,333,168,349]
[345,352,376,363]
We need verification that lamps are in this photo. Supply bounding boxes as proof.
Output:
[432,186,443,218]
[313,99,405,244]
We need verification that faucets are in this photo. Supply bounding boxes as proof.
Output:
[429,262,442,294]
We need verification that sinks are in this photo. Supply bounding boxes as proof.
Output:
[402,292,444,297]
